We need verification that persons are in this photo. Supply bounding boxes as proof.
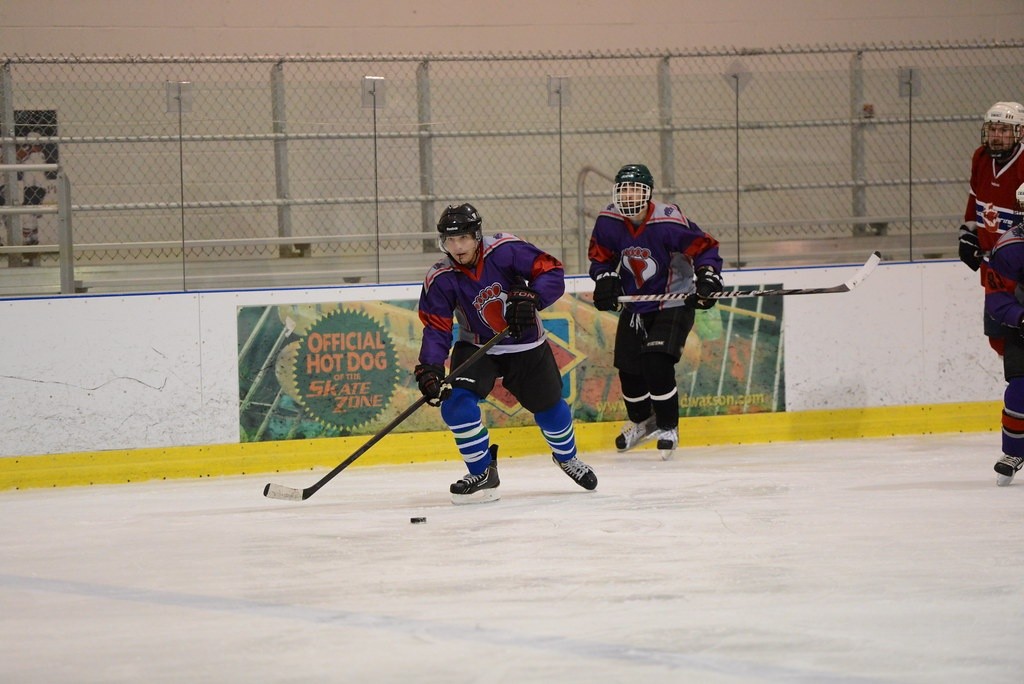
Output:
[589,164,723,462]
[959,101,1024,486]
[416,204,598,505]
[0,132,49,267]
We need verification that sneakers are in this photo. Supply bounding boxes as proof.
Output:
[615,412,660,453]
[994,455,1024,487]
[657,425,680,462]
[552,454,597,490]
[449,444,500,505]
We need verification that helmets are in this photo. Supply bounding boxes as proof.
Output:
[980,102,1024,162]
[436,202,484,245]
[612,163,654,218]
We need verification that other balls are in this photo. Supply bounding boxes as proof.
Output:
[410,517,426,523]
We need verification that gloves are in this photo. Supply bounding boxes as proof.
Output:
[683,266,723,310]
[593,269,621,312]
[503,290,539,342]
[958,223,985,271]
[413,364,453,406]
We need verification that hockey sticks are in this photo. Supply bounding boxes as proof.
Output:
[618,250,882,303]
[974,250,992,258]
[263,323,513,502]
